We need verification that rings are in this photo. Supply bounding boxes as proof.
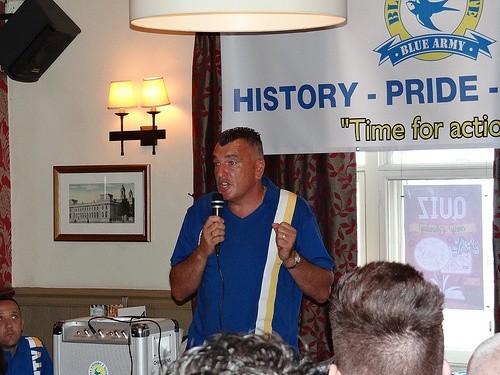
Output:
[211,232,215,238]
[282,233,286,239]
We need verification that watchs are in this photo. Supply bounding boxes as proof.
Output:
[285,253,302,269]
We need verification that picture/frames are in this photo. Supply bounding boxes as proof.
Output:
[52,163,153,244]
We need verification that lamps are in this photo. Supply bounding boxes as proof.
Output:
[128,0,348,35]
[107,76,171,156]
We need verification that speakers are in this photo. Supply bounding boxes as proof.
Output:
[0,0,81,83]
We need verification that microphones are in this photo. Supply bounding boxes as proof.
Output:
[211,193,224,256]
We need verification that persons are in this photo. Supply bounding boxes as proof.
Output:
[151,318,317,375]
[329,260,451,375]
[0,286,53,375]
[169,127,334,353]
[466,332,500,375]
